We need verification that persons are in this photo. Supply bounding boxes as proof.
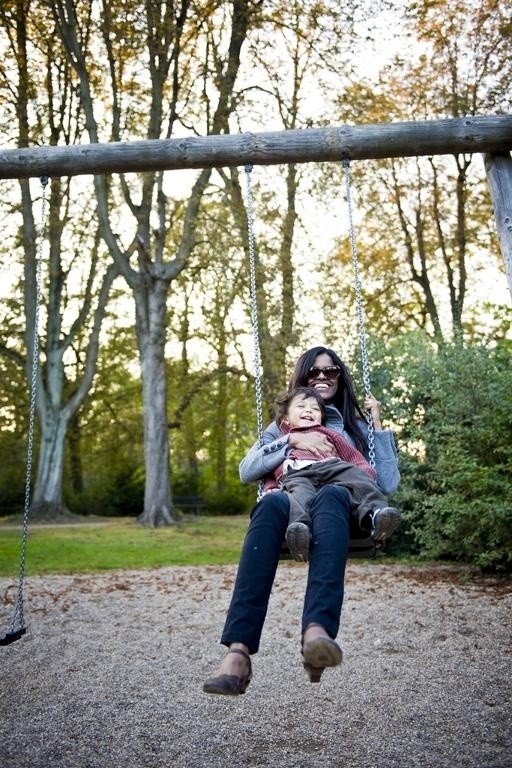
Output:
[260,386,401,564]
[201,347,401,694]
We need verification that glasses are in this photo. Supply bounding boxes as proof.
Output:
[308,365,341,378]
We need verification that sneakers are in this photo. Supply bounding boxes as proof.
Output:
[370,506,401,541]
[285,521,311,563]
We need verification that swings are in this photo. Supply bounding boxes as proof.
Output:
[0,176,48,648]
[242,152,380,560]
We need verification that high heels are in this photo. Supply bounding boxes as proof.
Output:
[302,623,343,683]
[203,648,253,696]
